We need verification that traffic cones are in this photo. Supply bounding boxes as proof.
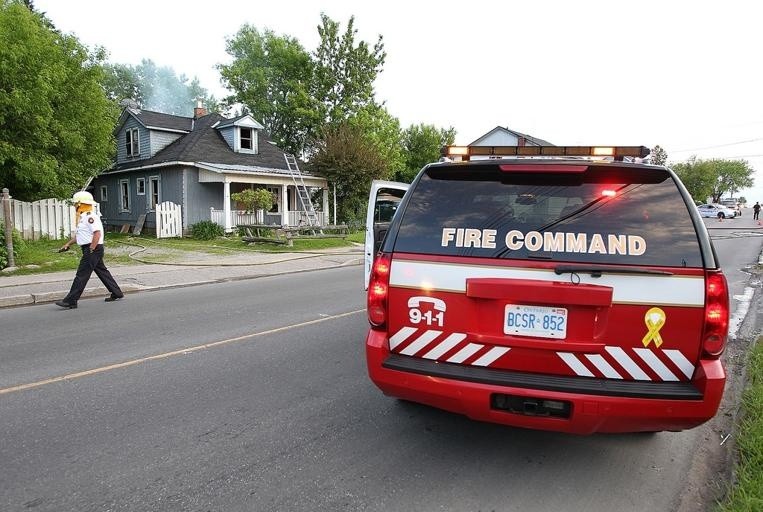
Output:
[717,214,724,223]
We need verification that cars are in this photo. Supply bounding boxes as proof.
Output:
[361,145,731,437]
[696,198,741,220]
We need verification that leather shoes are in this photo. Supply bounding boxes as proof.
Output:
[105,295,122,302]
[56,300,77,309]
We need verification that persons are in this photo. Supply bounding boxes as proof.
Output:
[51,190,123,309]
[752,201,761,221]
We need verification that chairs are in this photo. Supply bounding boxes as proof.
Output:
[472,200,513,219]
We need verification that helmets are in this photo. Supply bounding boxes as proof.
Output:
[69,191,93,205]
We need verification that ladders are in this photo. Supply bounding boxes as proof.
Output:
[284,153,325,237]
